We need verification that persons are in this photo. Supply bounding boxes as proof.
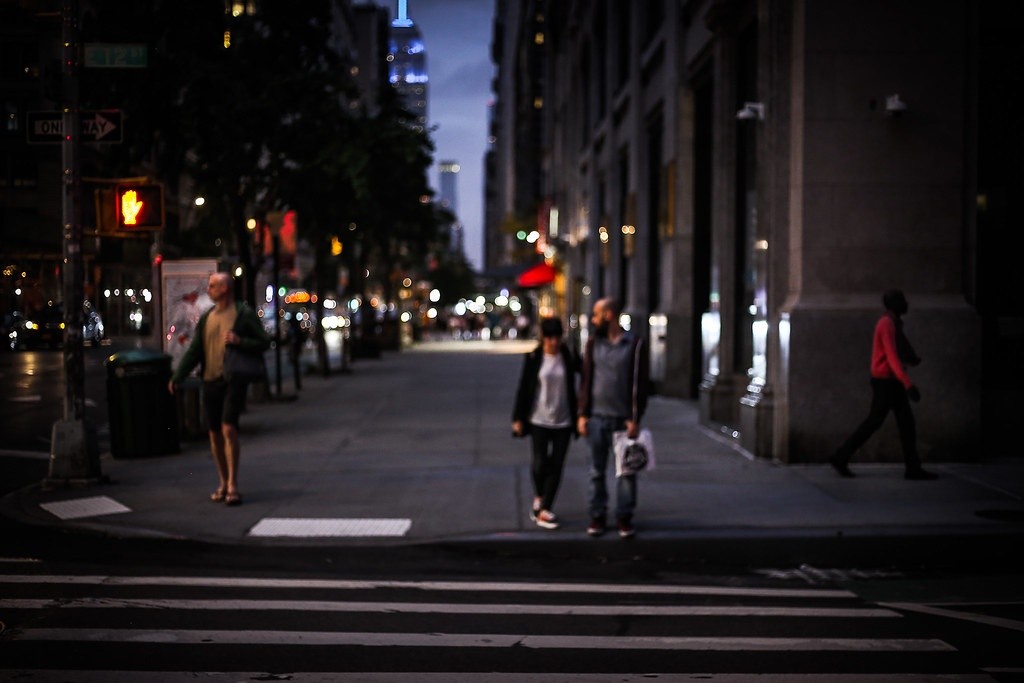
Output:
[577,297,648,537]
[511,318,578,530]
[168,272,270,505]
[829,289,923,481]
[281,311,306,398]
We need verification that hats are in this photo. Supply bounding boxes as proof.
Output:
[541,317,564,337]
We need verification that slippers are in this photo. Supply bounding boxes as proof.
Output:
[225,490,242,504]
[211,488,225,502]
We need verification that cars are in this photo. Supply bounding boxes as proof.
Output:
[35,299,105,352]
[0,310,35,353]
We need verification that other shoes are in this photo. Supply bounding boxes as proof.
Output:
[530,496,543,522]
[587,519,607,535]
[904,468,938,481]
[830,458,858,479]
[535,509,560,529]
[616,514,634,537]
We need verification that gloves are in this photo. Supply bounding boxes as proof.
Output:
[905,385,921,403]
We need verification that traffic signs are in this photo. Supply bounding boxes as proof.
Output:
[23,107,130,147]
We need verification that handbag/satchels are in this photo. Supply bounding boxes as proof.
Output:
[612,427,656,478]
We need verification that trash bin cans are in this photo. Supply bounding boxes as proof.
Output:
[107,348,179,458]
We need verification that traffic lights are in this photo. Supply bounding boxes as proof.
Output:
[101,181,167,233]
